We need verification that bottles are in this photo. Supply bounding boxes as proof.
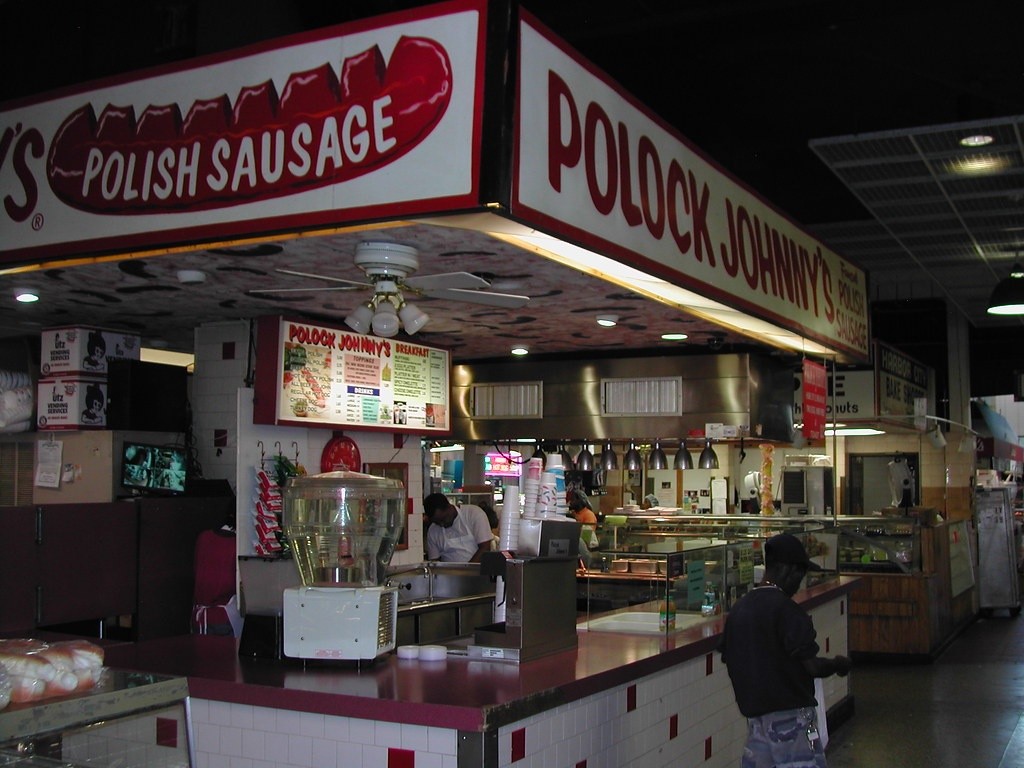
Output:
[659,596,675,633]
[700,580,722,618]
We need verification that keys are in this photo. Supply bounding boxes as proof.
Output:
[807,727,817,749]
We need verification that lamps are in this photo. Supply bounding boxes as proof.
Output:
[925,418,947,448]
[14,287,41,304]
[596,313,621,326]
[511,345,530,355]
[958,430,978,453]
[344,274,431,340]
[535,439,718,472]
[176,271,205,288]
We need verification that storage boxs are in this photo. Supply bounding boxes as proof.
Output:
[36,324,140,430]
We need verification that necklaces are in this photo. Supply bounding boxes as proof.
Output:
[760,581,784,592]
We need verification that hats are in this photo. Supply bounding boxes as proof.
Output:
[765,532,821,573]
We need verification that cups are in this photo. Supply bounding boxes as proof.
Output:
[498,452,568,554]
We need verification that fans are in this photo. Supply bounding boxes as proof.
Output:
[250,241,530,310]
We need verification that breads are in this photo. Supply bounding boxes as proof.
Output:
[0,637,105,704]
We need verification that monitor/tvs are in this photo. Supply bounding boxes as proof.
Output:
[121,440,190,497]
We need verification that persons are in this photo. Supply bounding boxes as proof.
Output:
[721,533,851,768]
[144,449,149,462]
[567,490,599,565]
[644,494,659,508]
[152,447,159,466]
[426,404,435,424]
[398,409,404,424]
[189,497,242,637]
[146,467,153,486]
[179,477,185,487]
[424,493,506,633]
[160,474,170,488]
